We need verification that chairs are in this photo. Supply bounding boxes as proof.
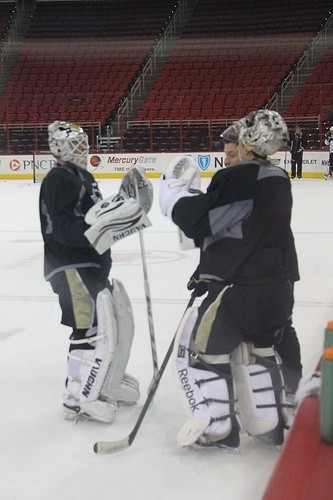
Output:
[0,0,333,150]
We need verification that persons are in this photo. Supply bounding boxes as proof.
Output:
[158,110,302,453]
[39,120,153,423]
[324,126,333,180]
[288,125,306,180]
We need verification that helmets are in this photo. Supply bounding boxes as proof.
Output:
[219,121,241,142]
[231,108,291,158]
[47,120,90,171]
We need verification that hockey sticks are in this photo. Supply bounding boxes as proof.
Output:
[93,290,197,455]
[119,167,158,380]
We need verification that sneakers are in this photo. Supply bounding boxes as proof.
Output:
[253,417,288,450]
[173,416,242,455]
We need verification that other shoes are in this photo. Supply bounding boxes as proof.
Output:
[297,175,302,178]
[290,176,295,179]
